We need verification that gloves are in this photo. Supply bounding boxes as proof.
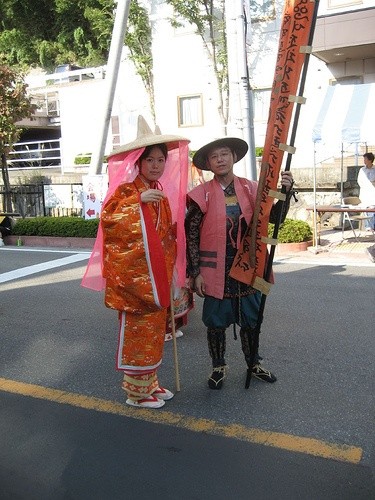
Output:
[280,170,294,195]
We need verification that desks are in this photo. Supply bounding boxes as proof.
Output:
[306,206,375,246]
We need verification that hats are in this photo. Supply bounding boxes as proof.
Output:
[105,115,191,164]
[192,129,249,172]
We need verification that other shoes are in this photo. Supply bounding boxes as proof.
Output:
[164,333,174,342]
[169,329,184,338]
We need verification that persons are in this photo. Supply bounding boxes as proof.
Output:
[357,153,375,232]
[154,125,194,342]
[185,138,294,390]
[0,210,11,247]
[80,115,192,408]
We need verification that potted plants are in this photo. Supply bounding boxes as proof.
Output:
[73,152,108,174]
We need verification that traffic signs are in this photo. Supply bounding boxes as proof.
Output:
[82,174,109,220]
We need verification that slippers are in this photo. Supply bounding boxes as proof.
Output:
[208,365,228,390]
[245,363,277,383]
[125,394,166,409]
[152,386,174,401]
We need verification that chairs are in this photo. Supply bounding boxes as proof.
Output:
[342,197,375,243]
